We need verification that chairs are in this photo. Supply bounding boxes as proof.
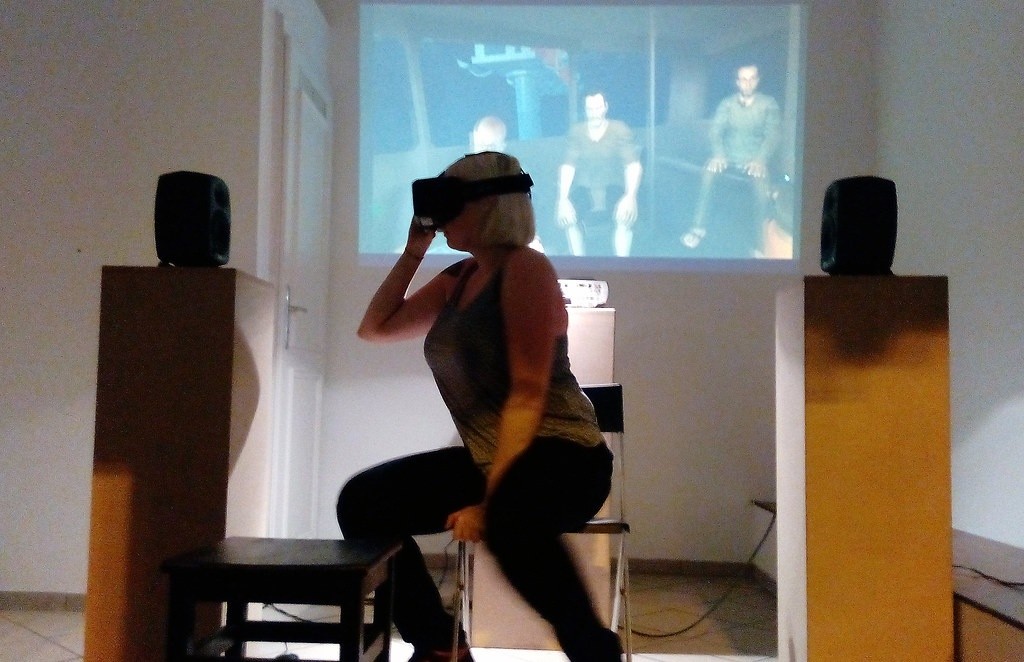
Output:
[450,383,632,662]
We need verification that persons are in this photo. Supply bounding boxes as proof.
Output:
[555,91,643,258]
[680,65,780,249]
[338,152,622,662]
[470,118,508,153]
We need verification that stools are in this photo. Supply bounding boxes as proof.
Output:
[159,536,402,662]
